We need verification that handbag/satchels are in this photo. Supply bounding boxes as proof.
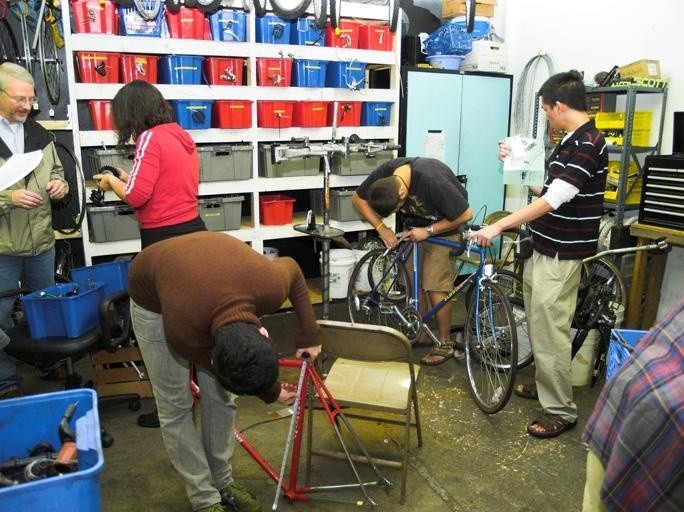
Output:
[48,194,79,230]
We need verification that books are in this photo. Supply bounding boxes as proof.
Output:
[0,148,45,197]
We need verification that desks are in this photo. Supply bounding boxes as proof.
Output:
[625,223,684,332]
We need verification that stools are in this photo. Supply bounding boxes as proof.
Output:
[0,288,140,449]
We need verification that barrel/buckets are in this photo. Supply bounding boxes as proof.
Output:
[512,283,626,387]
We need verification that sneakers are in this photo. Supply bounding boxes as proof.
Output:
[220,482,261,512]
[4,390,24,399]
[197,503,227,511]
[137,409,160,427]
[40,368,65,380]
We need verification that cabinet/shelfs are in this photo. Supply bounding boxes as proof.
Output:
[58,0,402,317]
[526,76,669,345]
[69,3,396,244]
[396,66,514,288]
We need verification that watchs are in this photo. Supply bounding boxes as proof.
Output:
[426,224,435,237]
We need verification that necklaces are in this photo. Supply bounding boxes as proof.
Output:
[392,174,409,193]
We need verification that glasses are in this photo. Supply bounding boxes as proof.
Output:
[1,89,39,104]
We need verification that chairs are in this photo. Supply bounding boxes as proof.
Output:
[305,318,424,506]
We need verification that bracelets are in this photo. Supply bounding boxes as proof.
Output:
[374,220,386,233]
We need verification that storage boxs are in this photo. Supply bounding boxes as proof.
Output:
[86,99,393,130]
[84,139,394,186]
[20,281,107,339]
[71,1,392,53]
[0,387,106,512]
[440,1,497,21]
[88,187,366,244]
[70,49,369,90]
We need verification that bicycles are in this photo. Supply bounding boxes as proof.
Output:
[368,175,629,373]
[346,225,526,414]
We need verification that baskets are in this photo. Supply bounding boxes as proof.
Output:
[423,23,509,73]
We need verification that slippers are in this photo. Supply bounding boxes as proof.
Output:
[421,339,454,364]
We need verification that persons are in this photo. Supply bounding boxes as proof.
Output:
[90,79,209,253]
[462,70,608,438]
[123,227,326,512]
[349,156,475,365]
[578,292,682,511]
[0,60,70,401]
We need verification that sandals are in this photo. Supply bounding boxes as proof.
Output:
[528,414,577,436]
[514,382,538,398]
